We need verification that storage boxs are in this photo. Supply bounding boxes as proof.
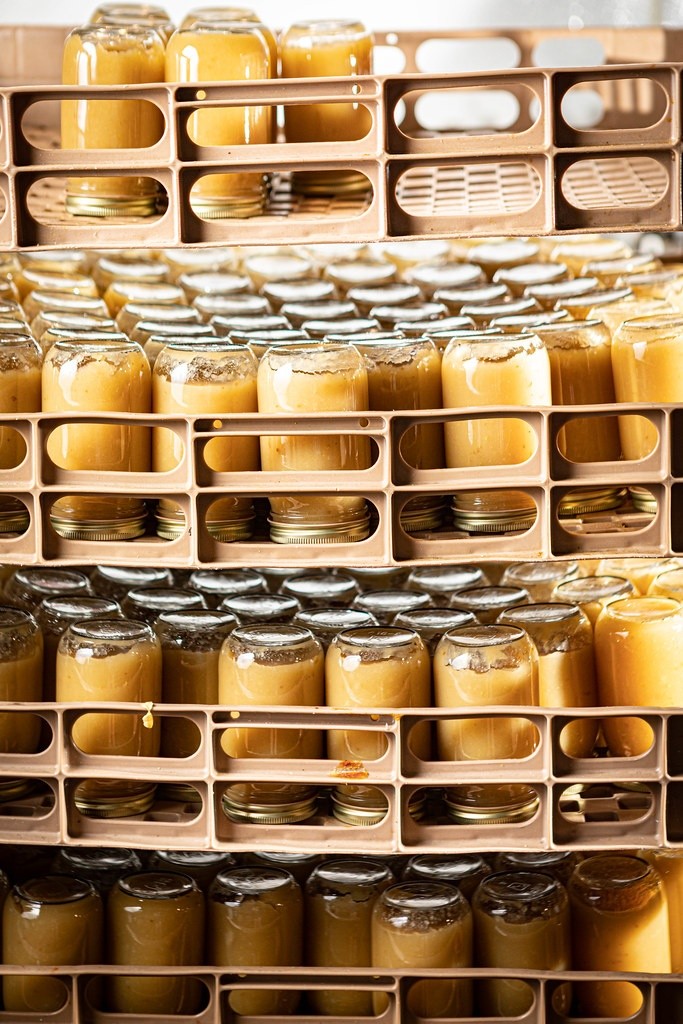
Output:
[0,694,683,856]
[0,25,683,254]
[0,961,683,1024]
[0,403,683,567]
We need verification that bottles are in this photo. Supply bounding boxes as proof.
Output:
[181,9,272,44]
[162,25,272,218]
[17,249,152,540]
[1,558,683,830]
[0,250,43,530]
[152,231,682,546]
[92,256,259,543]
[279,22,374,193]
[64,30,166,218]
[89,2,172,47]
[1,845,683,1024]
